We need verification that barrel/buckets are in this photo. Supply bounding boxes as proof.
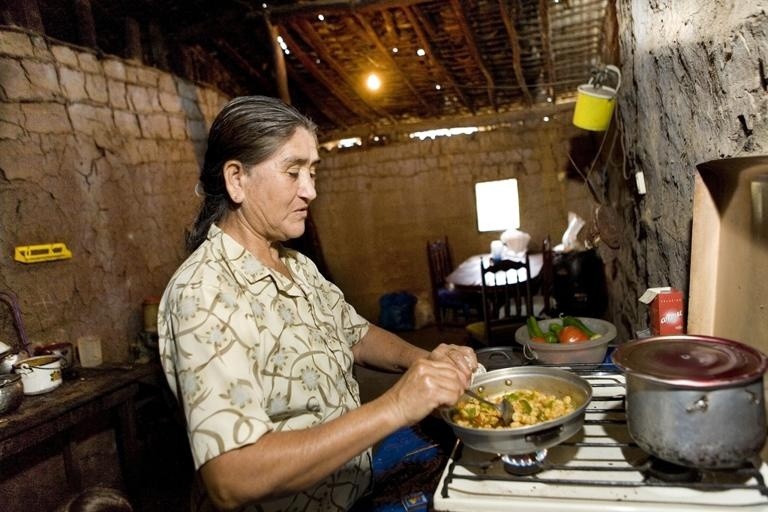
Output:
[12,353,64,398]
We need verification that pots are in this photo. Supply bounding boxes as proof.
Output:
[611,332,768,471]
[441,363,592,456]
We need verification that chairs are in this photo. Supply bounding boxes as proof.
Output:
[421,235,557,346]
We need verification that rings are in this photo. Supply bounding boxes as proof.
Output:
[447,347,456,355]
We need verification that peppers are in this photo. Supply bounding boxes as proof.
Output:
[527,312,602,343]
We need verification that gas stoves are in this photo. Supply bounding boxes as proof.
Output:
[430,372,767,511]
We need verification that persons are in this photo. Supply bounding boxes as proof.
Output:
[157,95,478,512]
[55,485,134,512]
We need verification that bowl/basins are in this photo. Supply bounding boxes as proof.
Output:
[513,318,615,369]
